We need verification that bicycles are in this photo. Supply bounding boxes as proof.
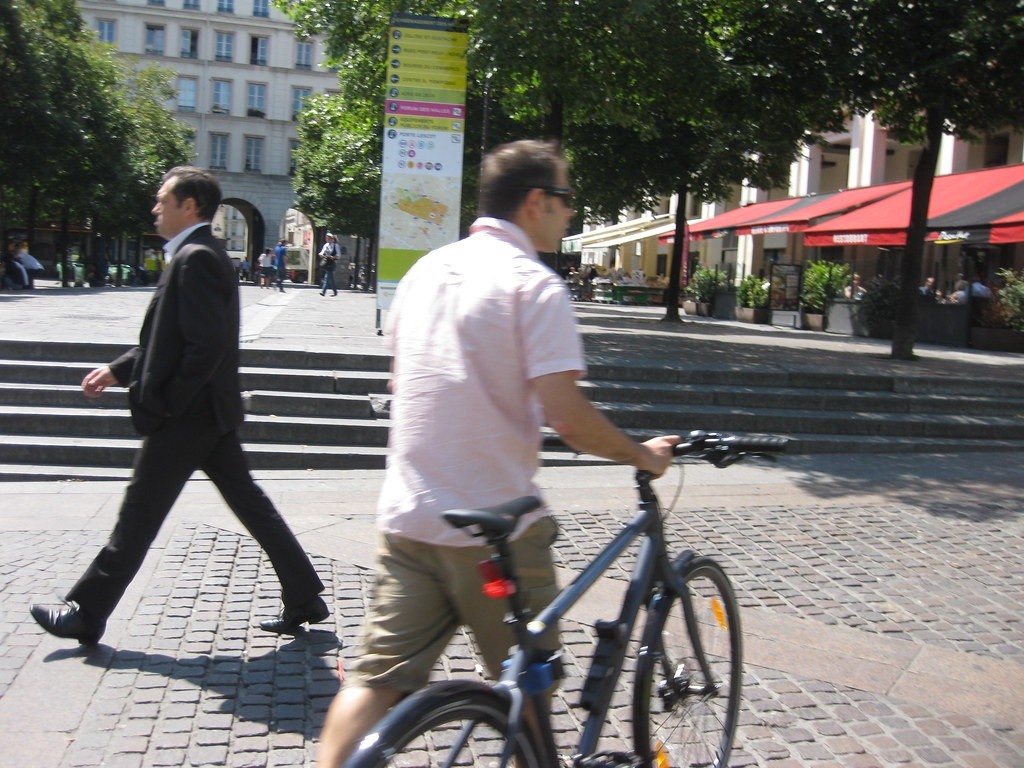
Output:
[336,429,797,768]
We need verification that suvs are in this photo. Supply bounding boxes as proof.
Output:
[270,243,310,284]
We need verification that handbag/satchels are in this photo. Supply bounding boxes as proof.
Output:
[320,251,330,266]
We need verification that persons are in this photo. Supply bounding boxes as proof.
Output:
[315,139,681,768]
[240,240,287,293]
[318,233,341,298]
[919,270,993,319]
[568,264,599,286]
[30,166,329,647]
[844,274,866,299]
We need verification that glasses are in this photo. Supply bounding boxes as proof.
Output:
[524,187,576,208]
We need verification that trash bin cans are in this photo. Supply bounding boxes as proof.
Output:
[108,264,130,284]
[57,262,85,282]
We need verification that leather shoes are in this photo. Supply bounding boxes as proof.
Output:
[30,600,106,645]
[260,597,330,632]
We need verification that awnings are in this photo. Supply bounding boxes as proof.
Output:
[659,163,1024,248]
[561,214,705,252]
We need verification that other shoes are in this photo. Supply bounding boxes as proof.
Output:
[280,290,286,293]
[320,293,324,296]
[334,291,337,296]
[273,283,276,289]
